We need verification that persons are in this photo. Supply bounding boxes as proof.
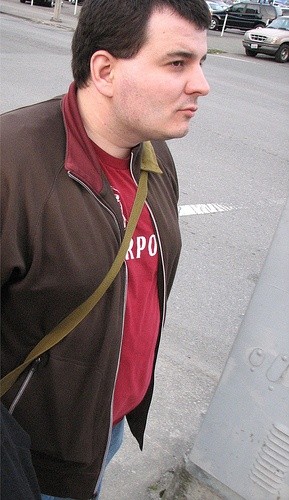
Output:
[2,0,214,500]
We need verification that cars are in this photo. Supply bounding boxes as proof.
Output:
[205,1,222,13]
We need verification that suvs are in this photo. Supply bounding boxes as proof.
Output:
[208,1,283,31]
[241,15,289,63]
